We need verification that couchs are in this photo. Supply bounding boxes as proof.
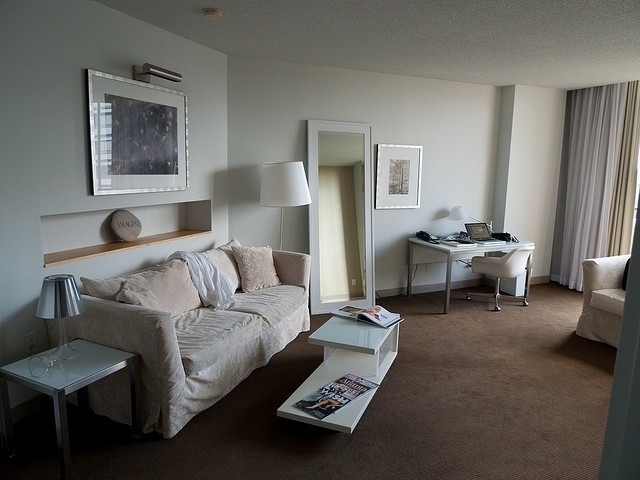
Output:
[576,255,630,348]
[47,240,311,438]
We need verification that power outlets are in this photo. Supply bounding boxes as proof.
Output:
[24,332,38,353]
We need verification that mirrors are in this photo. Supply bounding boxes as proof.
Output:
[305,119,376,317]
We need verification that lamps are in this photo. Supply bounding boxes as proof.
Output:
[259,161,312,251]
[36,275,87,363]
[133,62,183,84]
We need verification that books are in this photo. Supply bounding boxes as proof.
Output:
[441,241,478,247]
[330,305,404,329]
[294,373,380,421]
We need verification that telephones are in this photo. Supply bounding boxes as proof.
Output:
[416,231,439,241]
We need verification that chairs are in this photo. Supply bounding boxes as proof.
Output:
[467,244,535,311]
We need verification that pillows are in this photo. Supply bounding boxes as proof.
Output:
[232,245,282,292]
[199,236,241,308]
[116,280,162,312]
[79,258,200,313]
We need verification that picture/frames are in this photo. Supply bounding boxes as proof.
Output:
[85,68,190,195]
[376,143,423,209]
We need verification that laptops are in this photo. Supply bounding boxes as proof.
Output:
[464,223,506,245]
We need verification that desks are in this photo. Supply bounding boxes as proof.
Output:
[406,235,535,315]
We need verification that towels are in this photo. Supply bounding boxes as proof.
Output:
[168,250,235,310]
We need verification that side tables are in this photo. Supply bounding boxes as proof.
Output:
[0,338,141,480]
